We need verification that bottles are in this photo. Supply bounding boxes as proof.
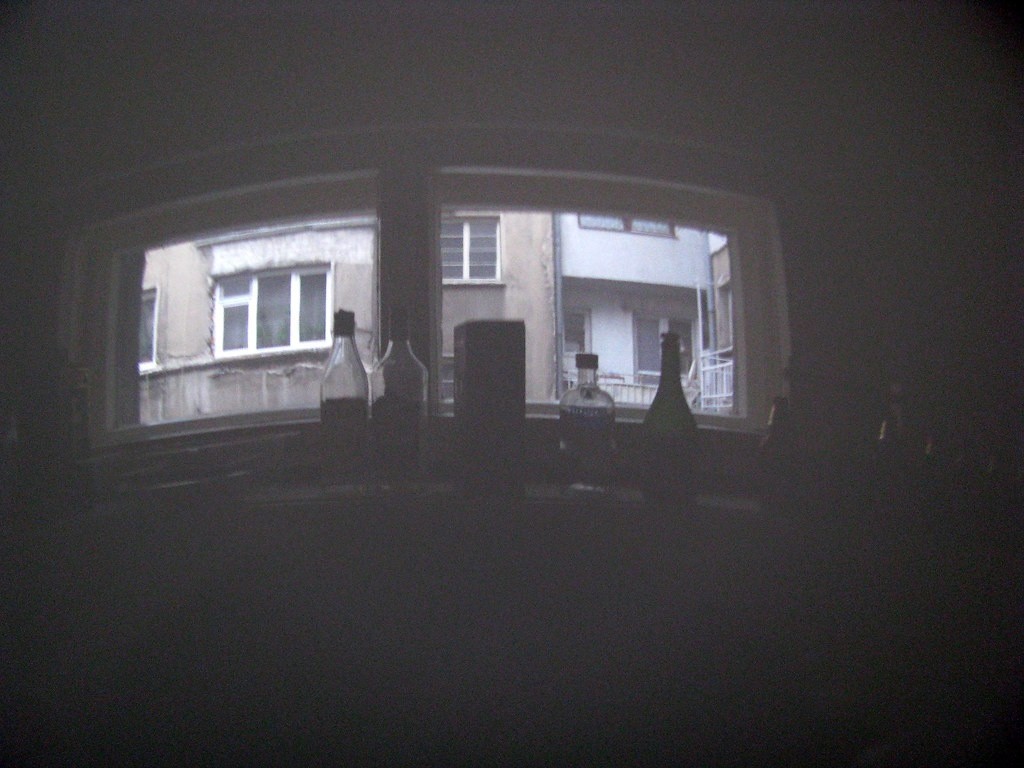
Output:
[370,316,429,417]
[559,354,615,418]
[643,333,698,428]
[320,310,368,423]
